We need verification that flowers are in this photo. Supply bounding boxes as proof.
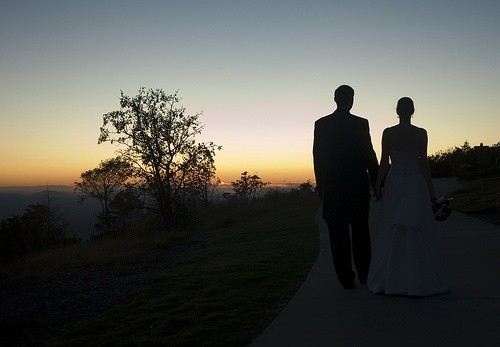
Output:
[431,200,452,222]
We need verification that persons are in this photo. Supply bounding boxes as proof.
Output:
[367,97,455,299]
[313,85,381,291]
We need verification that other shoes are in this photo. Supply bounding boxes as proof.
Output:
[343,282,357,290]
